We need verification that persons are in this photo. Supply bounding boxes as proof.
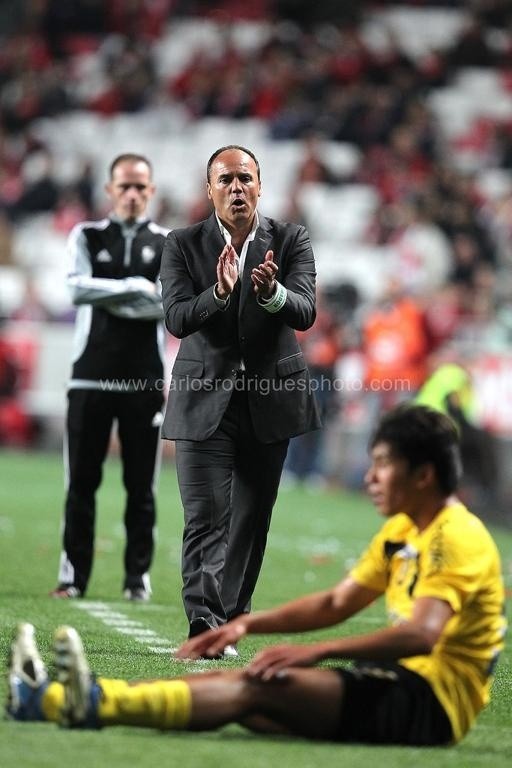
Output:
[44,155,173,598]
[5,401,508,750]
[156,143,328,660]
[1,0,511,528]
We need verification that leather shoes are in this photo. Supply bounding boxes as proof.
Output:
[188,617,225,660]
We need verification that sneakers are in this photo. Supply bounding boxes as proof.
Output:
[49,583,83,600]
[6,621,50,721]
[123,586,149,603]
[54,627,106,731]
